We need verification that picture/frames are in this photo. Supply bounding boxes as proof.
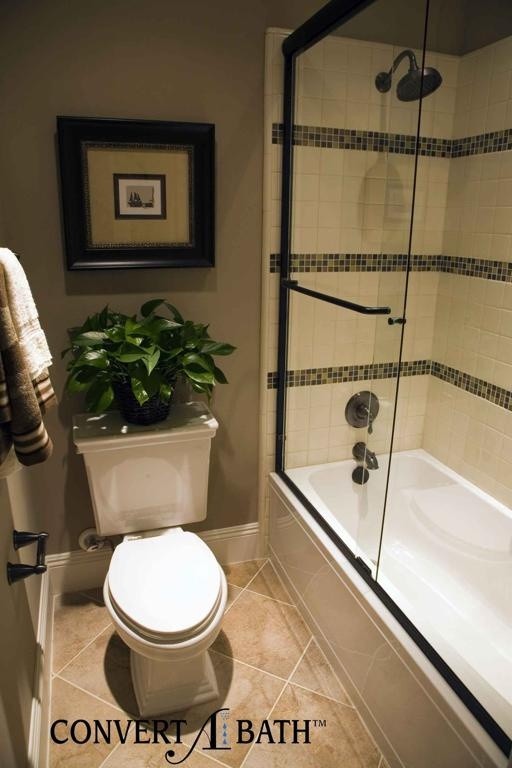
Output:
[55,114,218,271]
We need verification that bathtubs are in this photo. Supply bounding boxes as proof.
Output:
[264,450,512,767]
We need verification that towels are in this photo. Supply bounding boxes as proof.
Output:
[1,248,55,477]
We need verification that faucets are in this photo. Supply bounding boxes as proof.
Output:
[353,440,379,472]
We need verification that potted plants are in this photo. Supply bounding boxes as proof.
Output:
[58,298,239,427]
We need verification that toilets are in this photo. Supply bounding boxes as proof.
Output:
[69,406,229,720]
